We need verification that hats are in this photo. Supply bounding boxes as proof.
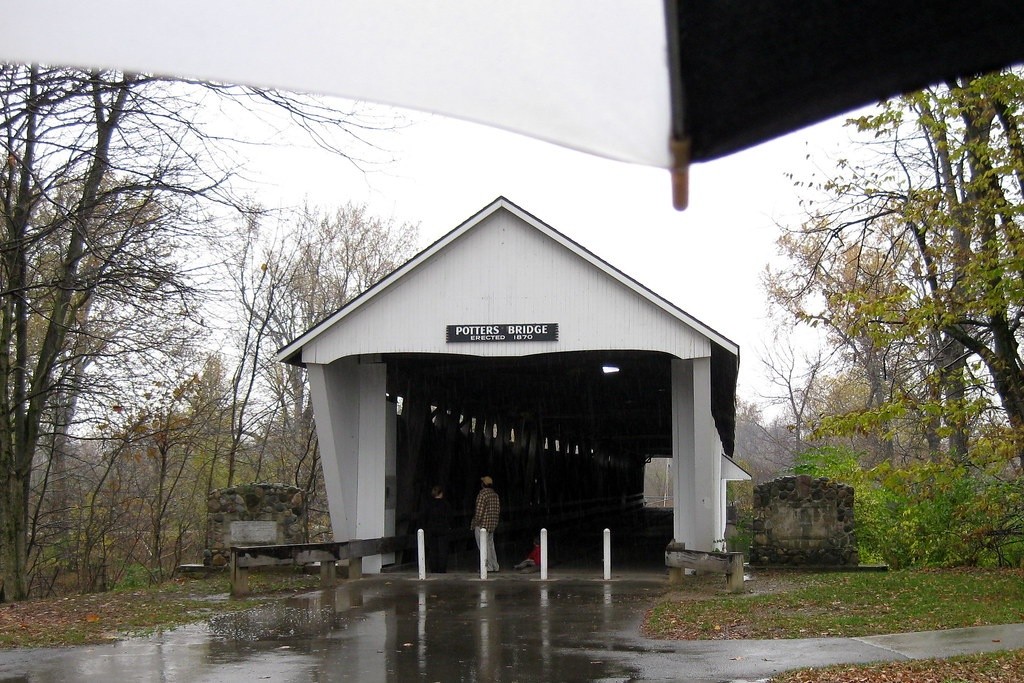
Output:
[480,476,493,485]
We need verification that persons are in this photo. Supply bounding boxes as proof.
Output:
[427,485,453,574]
[511,537,541,569]
[470,477,500,573]
